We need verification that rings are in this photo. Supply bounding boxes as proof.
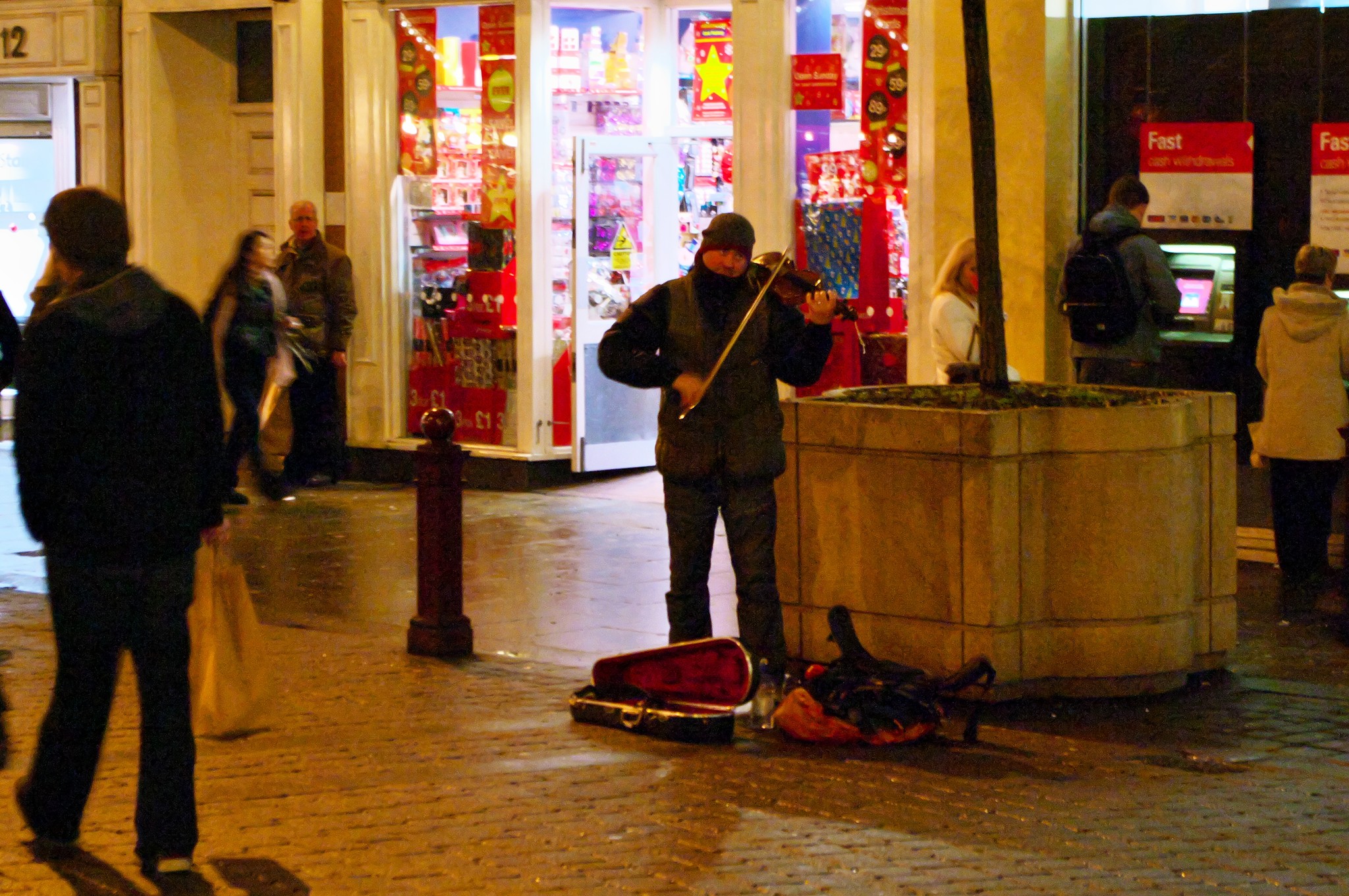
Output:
[816,301,821,305]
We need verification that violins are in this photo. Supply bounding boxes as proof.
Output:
[749,251,859,322]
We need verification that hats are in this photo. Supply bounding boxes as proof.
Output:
[701,212,754,266]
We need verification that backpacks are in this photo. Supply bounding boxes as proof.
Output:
[1064,229,1147,346]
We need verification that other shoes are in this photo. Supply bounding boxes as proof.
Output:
[144,852,192,875]
[15,773,78,848]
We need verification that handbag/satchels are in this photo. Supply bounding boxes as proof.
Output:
[191,540,272,734]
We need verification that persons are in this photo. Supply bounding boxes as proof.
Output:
[5,186,241,856]
[598,212,838,675]
[199,230,302,508]
[930,237,1020,387]
[1063,177,1181,390]
[1257,244,1349,595]
[266,202,359,488]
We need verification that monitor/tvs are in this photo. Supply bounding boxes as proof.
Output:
[1173,268,1215,316]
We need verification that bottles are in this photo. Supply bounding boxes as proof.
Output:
[751,663,776,729]
